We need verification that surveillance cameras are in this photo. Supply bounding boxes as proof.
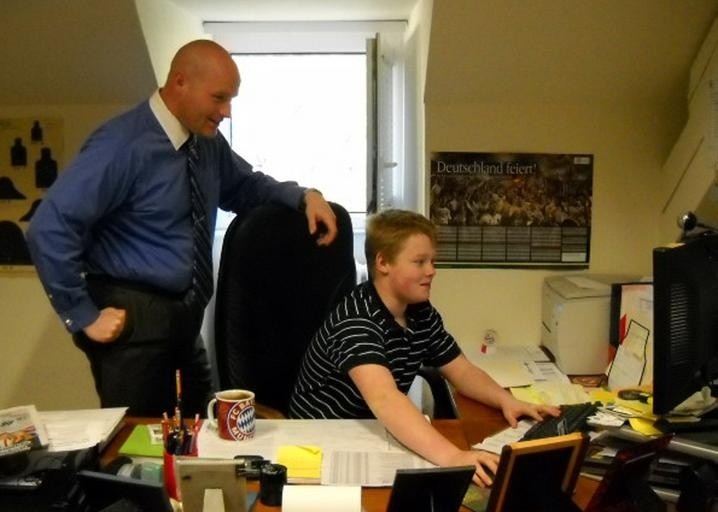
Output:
[677,212,715,234]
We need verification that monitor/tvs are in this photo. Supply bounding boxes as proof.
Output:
[652,233,718,417]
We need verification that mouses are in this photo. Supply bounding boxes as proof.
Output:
[466,452,509,492]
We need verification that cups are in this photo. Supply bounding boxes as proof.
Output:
[207,390,254,440]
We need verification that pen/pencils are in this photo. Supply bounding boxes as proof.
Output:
[162,368,199,453]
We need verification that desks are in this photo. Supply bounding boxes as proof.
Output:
[94,373,718,512]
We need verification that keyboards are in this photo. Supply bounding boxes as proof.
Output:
[518,401,603,442]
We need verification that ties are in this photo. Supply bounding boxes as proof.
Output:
[183,134,214,309]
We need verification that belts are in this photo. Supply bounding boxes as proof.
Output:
[87,272,186,300]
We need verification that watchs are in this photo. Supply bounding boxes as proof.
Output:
[301,188,323,206]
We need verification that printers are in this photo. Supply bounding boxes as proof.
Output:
[542,273,652,375]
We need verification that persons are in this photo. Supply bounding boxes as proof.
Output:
[26,39,341,418]
[431,176,592,227]
[285,207,563,492]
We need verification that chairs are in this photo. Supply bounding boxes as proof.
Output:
[214,200,458,419]
